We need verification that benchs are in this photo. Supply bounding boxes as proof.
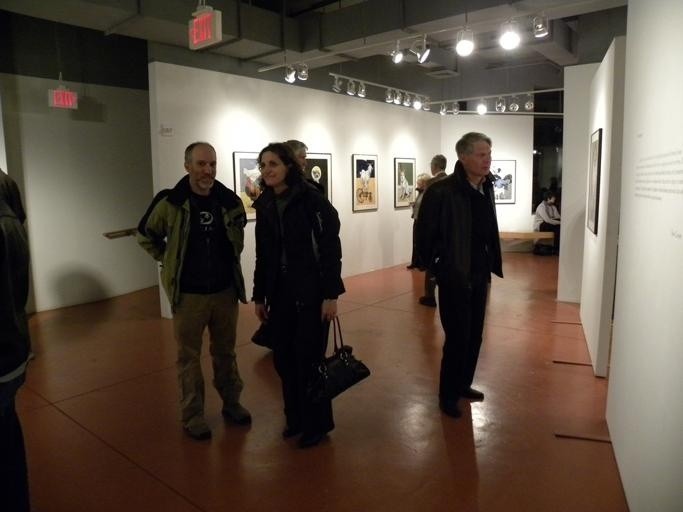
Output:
[499,231,553,252]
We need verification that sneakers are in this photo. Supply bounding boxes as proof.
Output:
[221,404,252,424]
[184,421,211,440]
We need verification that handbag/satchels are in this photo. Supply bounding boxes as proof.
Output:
[303,312,370,404]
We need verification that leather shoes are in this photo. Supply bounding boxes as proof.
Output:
[439,402,461,418]
[419,297,436,307]
[282,424,301,438]
[297,433,326,448]
[462,387,483,401]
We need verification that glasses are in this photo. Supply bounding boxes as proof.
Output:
[259,161,281,170]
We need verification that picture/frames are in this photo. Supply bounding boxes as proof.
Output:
[304,153,332,205]
[233,151,261,220]
[352,154,379,211]
[490,160,516,204]
[394,157,416,208]
[587,127,602,235]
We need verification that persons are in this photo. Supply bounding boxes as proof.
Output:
[248,143,348,449]
[283,138,309,172]
[135,141,253,442]
[532,189,561,257]
[419,154,448,308]
[406,172,430,272]
[0,167,33,511]
[548,176,559,193]
[417,131,503,420]
